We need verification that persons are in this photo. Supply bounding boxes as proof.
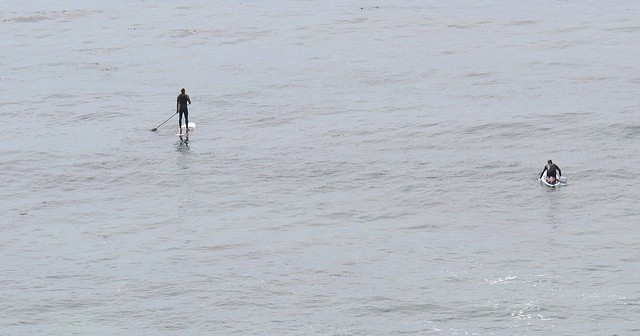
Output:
[177,88,191,131]
[540,160,561,184]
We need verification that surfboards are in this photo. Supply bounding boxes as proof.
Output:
[175,122,196,135]
[542,170,560,187]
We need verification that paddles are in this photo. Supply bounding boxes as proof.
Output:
[150,103,190,132]
